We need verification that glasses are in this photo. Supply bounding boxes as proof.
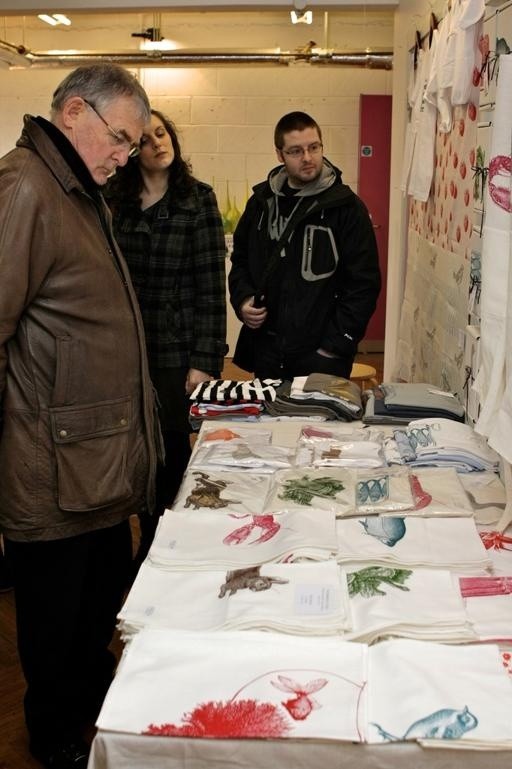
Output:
[278,141,324,159]
[80,97,143,161]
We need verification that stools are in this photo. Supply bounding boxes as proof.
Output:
[350,363,378,390]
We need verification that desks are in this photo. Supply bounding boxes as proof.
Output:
[85,417,512,769]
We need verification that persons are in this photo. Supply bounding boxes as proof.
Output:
[228,111,382,385]
[0,65,166,768]
[98,109,226,573]
